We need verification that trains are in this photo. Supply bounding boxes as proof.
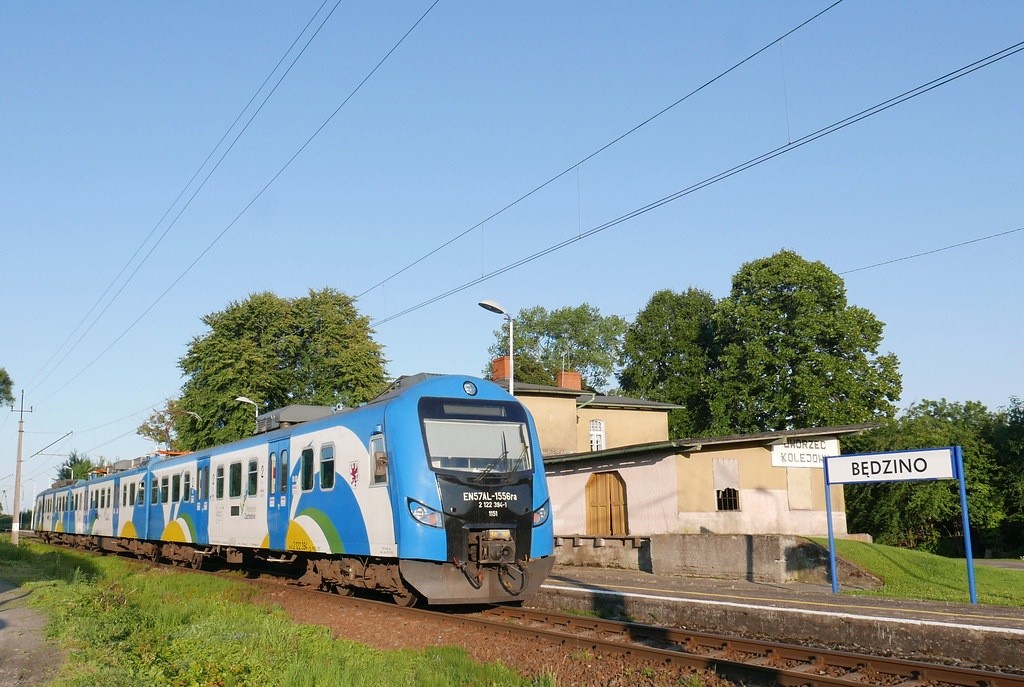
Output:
[30,372,558,607]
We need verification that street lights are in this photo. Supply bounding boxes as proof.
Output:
[478,300,514,396]
[63,465,74,481]
[236,396,258,435]
